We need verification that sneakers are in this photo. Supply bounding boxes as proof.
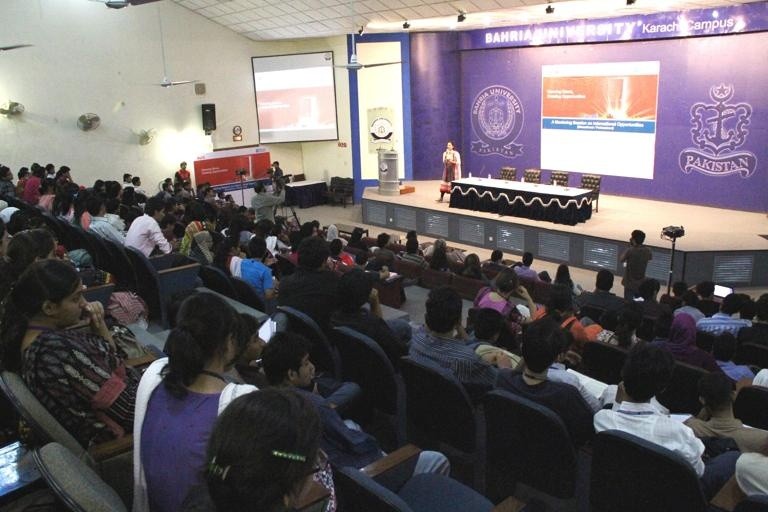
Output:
[434,198,444,203]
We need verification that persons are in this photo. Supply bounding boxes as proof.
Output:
[0,161,284,259]
[214,213,465,349]
[620,230,652,299]
[436,142,461,202]
[144,314,449,511]
[410,240,768,511]
[1,207,143,437]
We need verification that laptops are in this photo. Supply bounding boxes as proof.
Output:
[712,284,734,303]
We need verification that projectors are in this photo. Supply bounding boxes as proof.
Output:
[662,225,684,238]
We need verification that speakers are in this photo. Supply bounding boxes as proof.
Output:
[202,103,216,131]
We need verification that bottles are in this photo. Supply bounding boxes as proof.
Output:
[552,179,557,190]
[468,172,471,180]
[487,175,491,183]
[520,177,524,187]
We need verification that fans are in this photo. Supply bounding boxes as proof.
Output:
[75,112,102,133]
[129,1,203,87]
[0,41,35,53]
[133,126,158,145]
[0,100,25,118]
[88,0,167,10]
[324,29,408,71]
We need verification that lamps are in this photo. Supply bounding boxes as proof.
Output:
[357,0,639,37]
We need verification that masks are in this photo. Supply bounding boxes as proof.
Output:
[284,460,336,511]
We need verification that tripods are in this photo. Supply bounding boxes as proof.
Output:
[272,188,301,230]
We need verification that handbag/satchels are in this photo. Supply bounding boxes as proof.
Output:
[105,290,152,332]
